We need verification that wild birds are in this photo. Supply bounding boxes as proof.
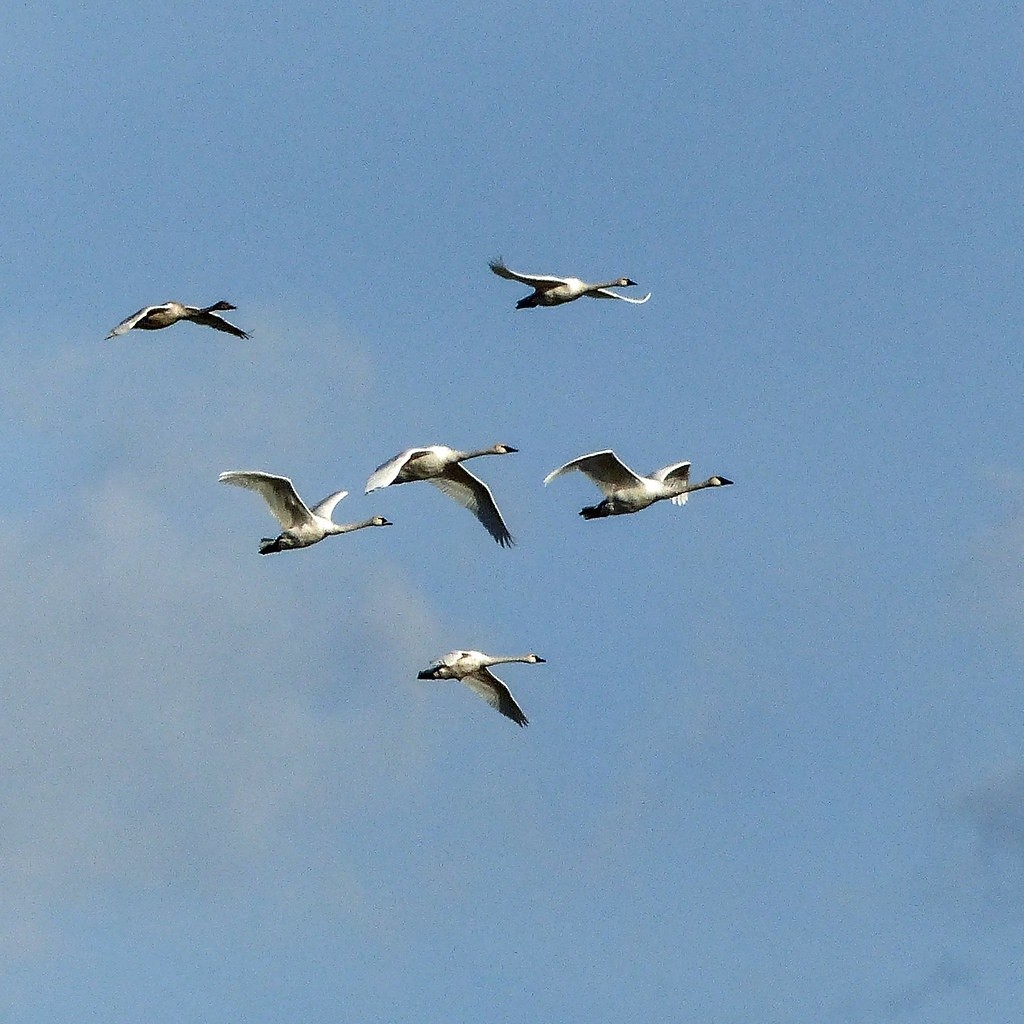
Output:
[363,442,519,552]
[488,250,653,311]
[217,469,394,557]
[416,647,547,730]
[102,300,256,344]
[543,449,738,521]
